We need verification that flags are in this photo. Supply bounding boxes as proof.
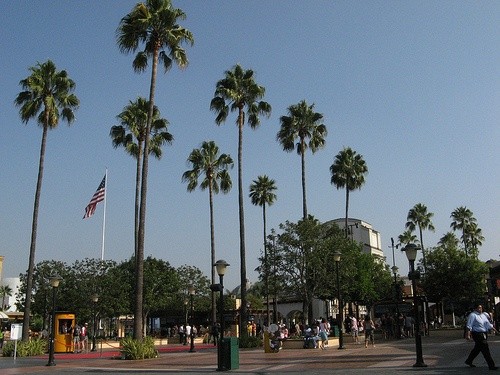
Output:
[82,174,105,220]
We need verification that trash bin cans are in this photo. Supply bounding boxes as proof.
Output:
[331,326,339,336]
[216,336,239,370]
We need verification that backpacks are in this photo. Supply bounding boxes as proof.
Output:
[74,328,80,336]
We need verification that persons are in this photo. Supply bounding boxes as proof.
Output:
[381,313,414,340]
[85,322,91,353]
[317,316,330,351]
[184,323,191,345]
[72,321,81,353]
[311,324,320,348]
[0,329,4,350]
[213,322,221,346]
[349,313,376,348]
[62,321,67,334]
[295,322,301,336]
[178,324,184,343]
[464,303,498,370]
[303,325,312,349]
[267,322,288,350]
[80,323,86,354]
[290,322,297,336]
[193,324,197,338]
[247,321,257,337]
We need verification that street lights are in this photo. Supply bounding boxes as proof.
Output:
[46,274,63,366]
[400,242,428,367]
[188,287,197,353]
[213,259,230,371]
[391,266,402,340]
[91,294,100,352]
[183,299,189,346]
[331,251,346,349]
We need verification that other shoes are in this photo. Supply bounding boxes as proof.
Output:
[90,348,97,352]
[464,359,475,367]
[320,345,327,350]
[488,363,498,370]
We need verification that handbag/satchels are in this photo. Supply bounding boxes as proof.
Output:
[369,320,376,330]
